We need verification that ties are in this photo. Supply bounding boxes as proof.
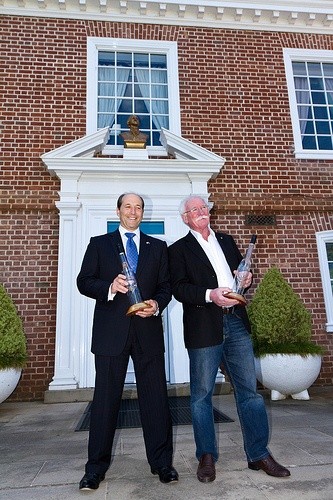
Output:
[125,233,139,273]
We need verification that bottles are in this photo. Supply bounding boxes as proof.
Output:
[231,235,258,295]
[116,243,143,304]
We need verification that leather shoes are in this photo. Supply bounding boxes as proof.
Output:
[197,453,216,483]
[248,455,291,477]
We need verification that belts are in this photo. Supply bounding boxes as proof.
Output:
[222,306,236,314]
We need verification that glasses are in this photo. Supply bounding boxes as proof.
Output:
[184,206,209,214]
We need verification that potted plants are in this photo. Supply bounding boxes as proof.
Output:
[245,266,324,401]
[0,285,30,404]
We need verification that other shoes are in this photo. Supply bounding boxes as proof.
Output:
[79,474,105,491]
[158,467,179,484]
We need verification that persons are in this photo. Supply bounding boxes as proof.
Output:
[119,115,149,142]
[167,195,291,483]
[75,193,179,491]
